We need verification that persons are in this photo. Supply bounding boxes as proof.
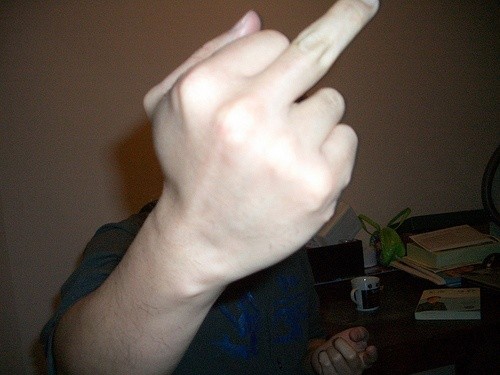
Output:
[40,0,383,375]
[416,290,448,312]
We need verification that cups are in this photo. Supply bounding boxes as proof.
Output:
[351,276,379,311]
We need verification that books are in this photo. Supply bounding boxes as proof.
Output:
[414,287,481,320]
[392,224,500,292]
